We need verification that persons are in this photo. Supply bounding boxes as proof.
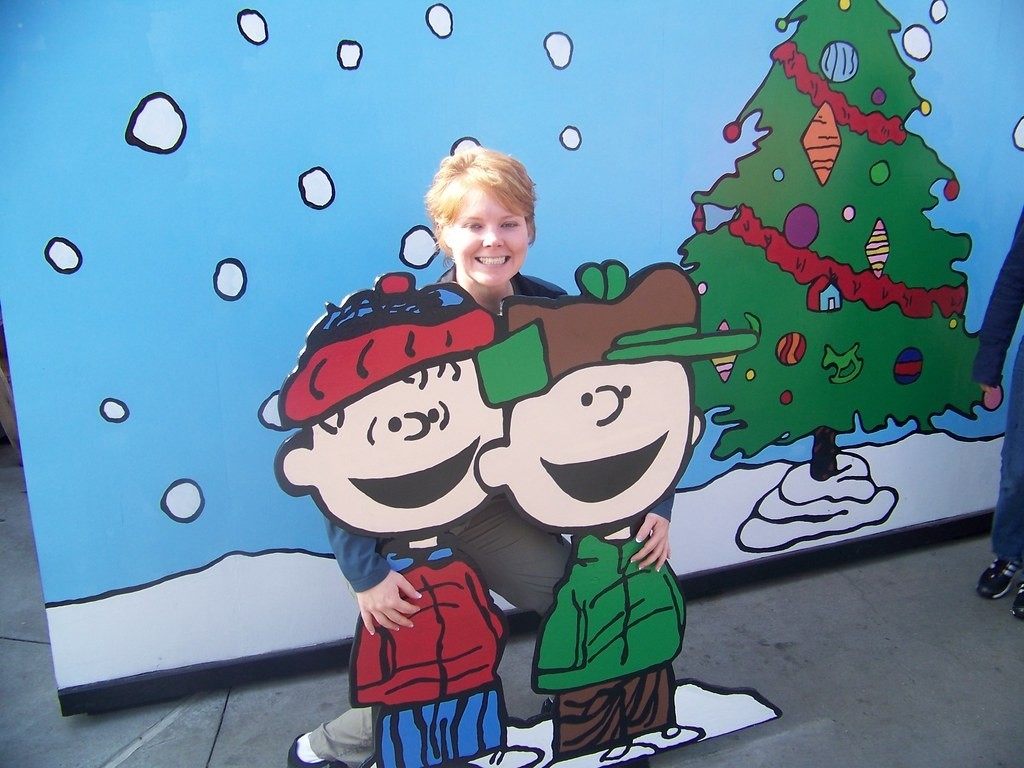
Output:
[286,147,676,768]
[971,205,1024,619]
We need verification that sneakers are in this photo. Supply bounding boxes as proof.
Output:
[1012,580,1024,618]
[976,558,1024,598]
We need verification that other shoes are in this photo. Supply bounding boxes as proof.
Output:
[288,734,346,768]
